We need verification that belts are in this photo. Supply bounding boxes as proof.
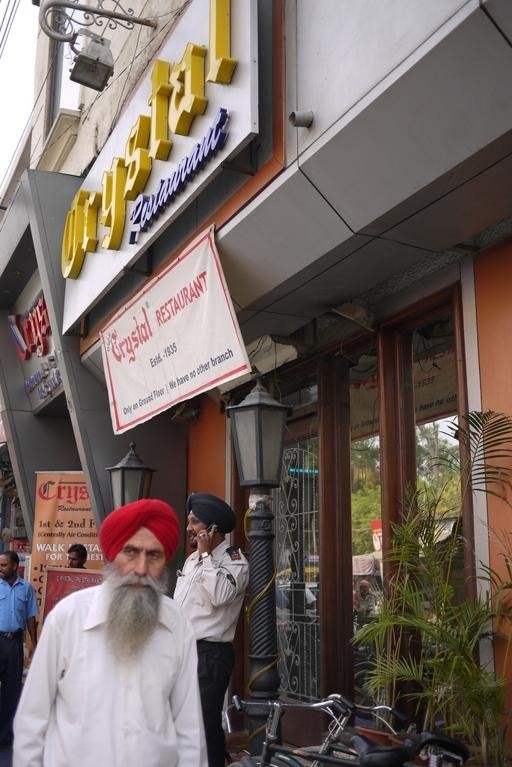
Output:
[0,630,24,640]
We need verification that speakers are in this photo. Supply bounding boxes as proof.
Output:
[325,345,361,375]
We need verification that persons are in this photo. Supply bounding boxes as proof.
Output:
[67,543,88,568]
[10,493,212,766]
[1,549,41,766]
[170,490,252,765]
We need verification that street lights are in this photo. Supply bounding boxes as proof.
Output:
[222,373,299,755]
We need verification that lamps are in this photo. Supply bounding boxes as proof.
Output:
[38,0,163,89]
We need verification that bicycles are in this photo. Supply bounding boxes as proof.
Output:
[217,691,477,766]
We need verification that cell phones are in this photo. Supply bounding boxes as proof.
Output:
[194,521,220,546]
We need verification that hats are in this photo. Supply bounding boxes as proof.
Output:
[97,499,181,565]
[359,579,367,588]
[185,491,235,534]
